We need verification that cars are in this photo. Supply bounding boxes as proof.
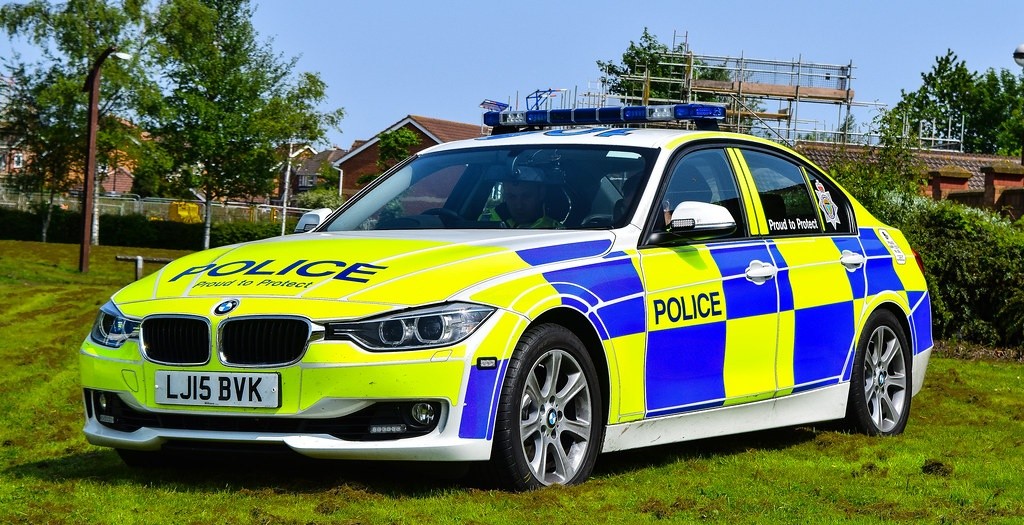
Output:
[77,105,936,493]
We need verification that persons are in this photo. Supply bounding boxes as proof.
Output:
[477,180,562,229]
[621,170,672,226]
